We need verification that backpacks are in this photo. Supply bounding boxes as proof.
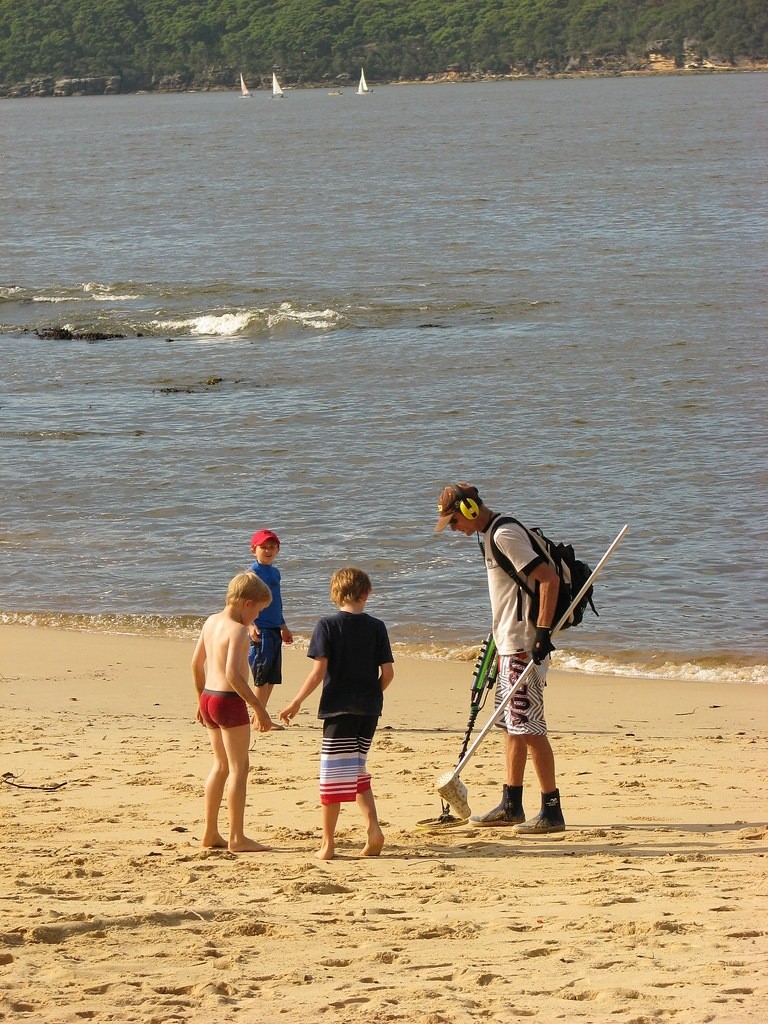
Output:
[490,516,600,630]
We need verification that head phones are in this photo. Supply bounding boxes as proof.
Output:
[449,483,479,520]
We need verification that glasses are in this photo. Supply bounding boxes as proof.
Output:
[448,511,462,526]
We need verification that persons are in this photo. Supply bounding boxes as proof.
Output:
[246,530,293,730]
[281,567,394,860]
[192,572,272,851]
[434,482,566,834]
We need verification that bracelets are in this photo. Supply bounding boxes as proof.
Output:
[536,626,550,629]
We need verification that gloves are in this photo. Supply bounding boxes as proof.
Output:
[529,625,556,666]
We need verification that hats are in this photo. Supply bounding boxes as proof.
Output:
[433,482,478,532]
[250,529,280,551]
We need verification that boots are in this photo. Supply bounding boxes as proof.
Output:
[512,788,566,834]
[469,784,526,827]
